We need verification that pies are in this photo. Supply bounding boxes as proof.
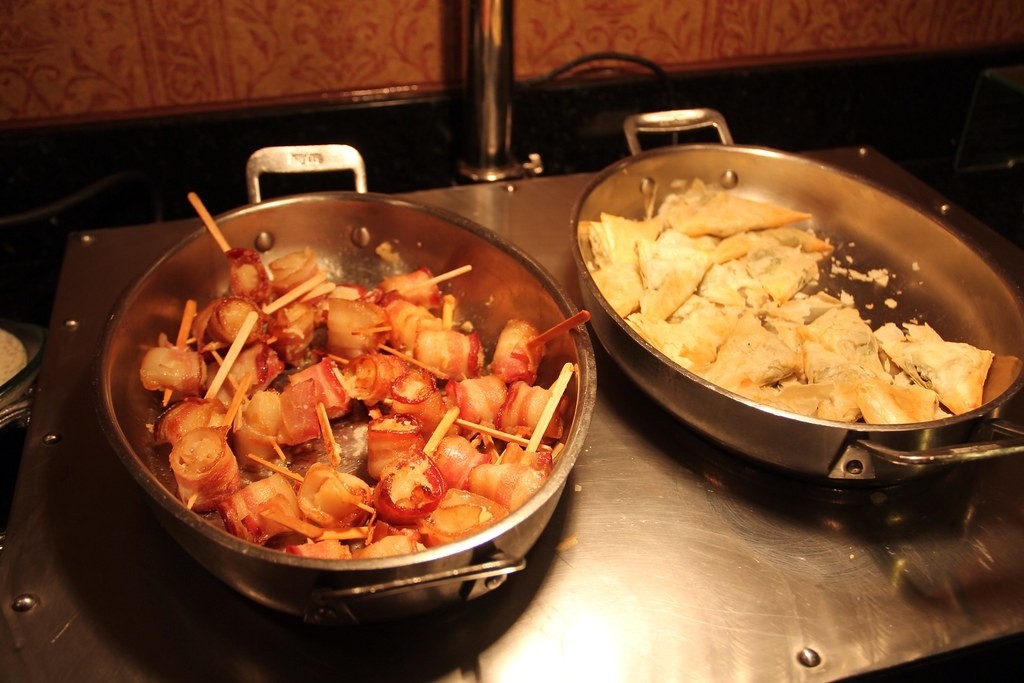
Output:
[583,180,994,426]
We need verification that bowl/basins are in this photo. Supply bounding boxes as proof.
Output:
[0,318,47,434]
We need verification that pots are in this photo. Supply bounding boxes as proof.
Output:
[93,145,598,623]
[570,107,1023,492]
